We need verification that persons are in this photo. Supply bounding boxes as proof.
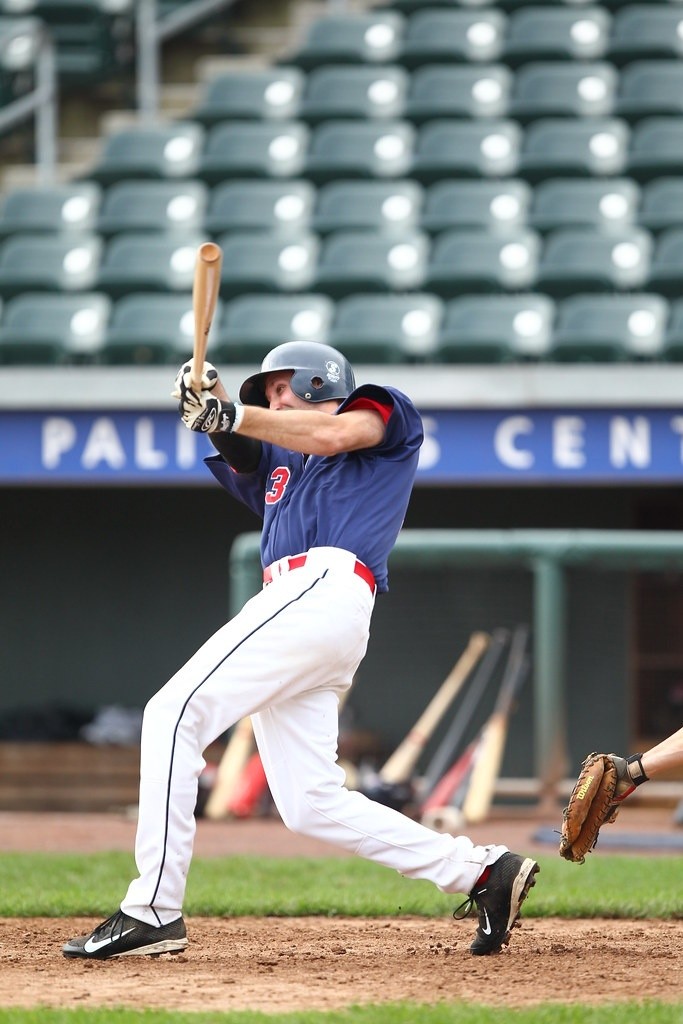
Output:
[559,726,683,863]
[62,341,538,963]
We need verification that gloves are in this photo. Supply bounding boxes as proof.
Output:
[171,358,219,399]
[172,389,244,434]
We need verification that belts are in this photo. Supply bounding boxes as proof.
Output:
[263,555,375,595]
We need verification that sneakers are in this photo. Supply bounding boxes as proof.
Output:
[62,909,189,959]
[453,852,540,956]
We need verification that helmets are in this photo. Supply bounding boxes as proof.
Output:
[239,341,356,404]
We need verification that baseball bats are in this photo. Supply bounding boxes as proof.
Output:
[191,241,225,397]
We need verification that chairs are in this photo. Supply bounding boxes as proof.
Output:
[0,1,683,362]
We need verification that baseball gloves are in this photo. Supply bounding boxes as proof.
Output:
[551,751,637,866]
